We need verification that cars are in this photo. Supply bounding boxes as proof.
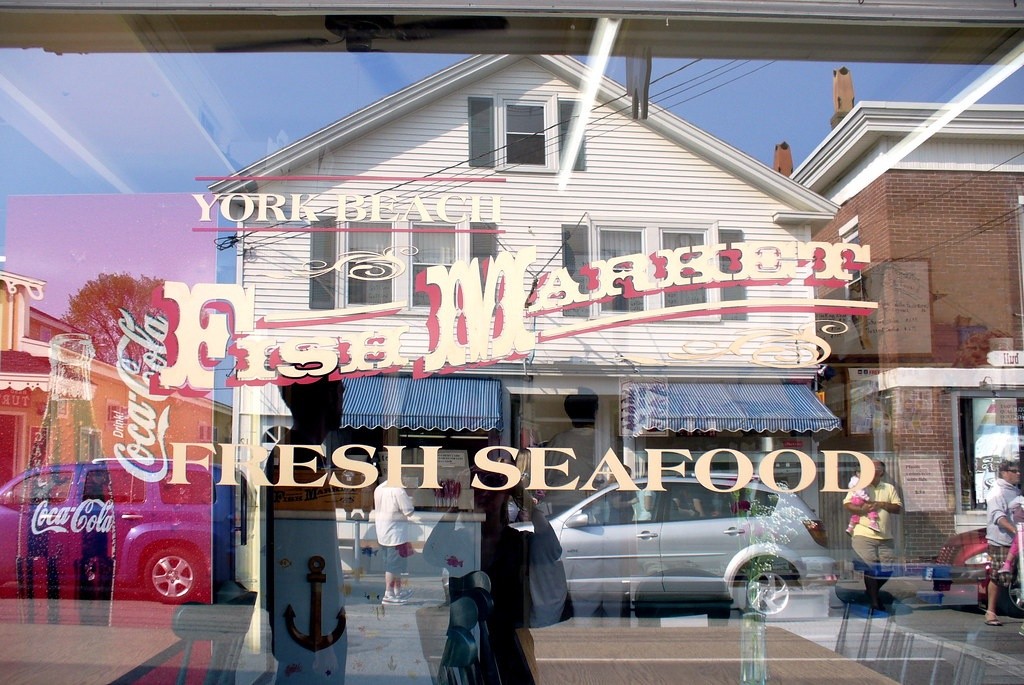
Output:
[933,524,1024,616]
[0,460,256,604]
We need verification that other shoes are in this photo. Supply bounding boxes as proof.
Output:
[873,604,885,610]
[983,613,1001,625]
[997,564,1011,573]
[1019,625,1024,636]
[846,527,853,537]
[397,591,415,599]
[869,521,882,533]
[382,593,408,606]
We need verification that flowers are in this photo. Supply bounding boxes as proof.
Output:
[730,473,817,677]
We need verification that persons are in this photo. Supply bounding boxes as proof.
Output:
[424,449,562,685]
[259,357,349,685]
[544,385,616,621]
[843,461,903,612]
[373,473,414,605]
[606,464,722,526]
[984,460,1024,626]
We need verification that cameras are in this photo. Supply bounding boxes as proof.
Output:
[480,472,502,492]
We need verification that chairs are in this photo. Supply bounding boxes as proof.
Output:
[417,569,511,685]
[171,577,258,685]
[831,585,1024,685]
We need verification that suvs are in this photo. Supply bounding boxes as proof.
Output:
[442,473,840,621]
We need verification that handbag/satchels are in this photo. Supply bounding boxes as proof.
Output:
[520,533,574,629]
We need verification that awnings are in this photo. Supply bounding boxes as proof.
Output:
[622,382,843,433]
[332,375,503,431]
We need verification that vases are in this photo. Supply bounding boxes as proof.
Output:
[741,611,770,685]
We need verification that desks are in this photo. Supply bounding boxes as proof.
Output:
[515,624,899,685]
[0,620,186,685]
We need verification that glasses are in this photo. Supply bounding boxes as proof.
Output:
[1004,469,1021,473]
